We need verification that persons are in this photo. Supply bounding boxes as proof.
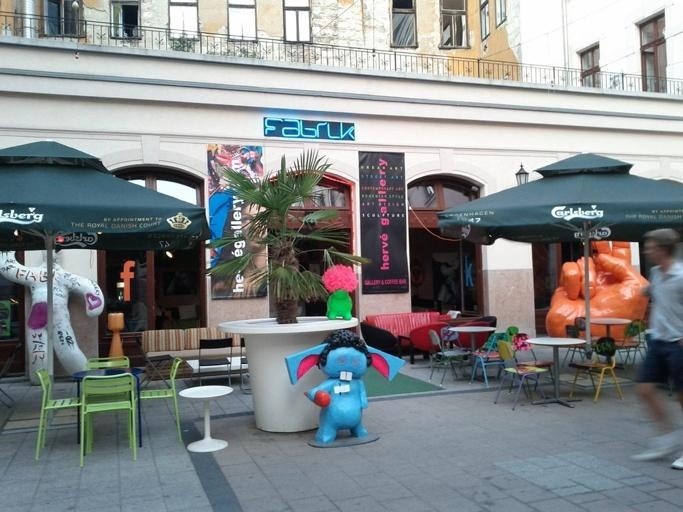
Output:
[628,229,682,469]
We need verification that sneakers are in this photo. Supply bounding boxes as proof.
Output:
[633,436,683,460]
[670,454,683,469]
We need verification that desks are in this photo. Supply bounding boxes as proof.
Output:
[178,383,236,454]
[74,367,145,453]
[182,355,249,389]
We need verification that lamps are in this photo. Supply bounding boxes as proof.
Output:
[514,162,530,185]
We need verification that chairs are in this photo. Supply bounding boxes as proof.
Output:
[34,366,94,460]
[129,355,186,447]
[78,370,138,469]
[84,355,130,376]
[196,337,233,388]
[238,335,252,396]
[130,333,173,392]
[364,308,652,410]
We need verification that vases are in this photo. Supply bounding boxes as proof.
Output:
[324,288,353,321]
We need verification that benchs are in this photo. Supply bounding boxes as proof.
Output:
[139,325,252,382]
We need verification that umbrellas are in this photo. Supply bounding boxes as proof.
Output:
[437,152,683,360]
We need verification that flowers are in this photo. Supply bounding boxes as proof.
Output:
[320,263,359,295]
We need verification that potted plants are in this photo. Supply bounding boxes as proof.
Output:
[199,145,373,436]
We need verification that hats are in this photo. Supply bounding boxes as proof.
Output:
[643,228,681,245]
